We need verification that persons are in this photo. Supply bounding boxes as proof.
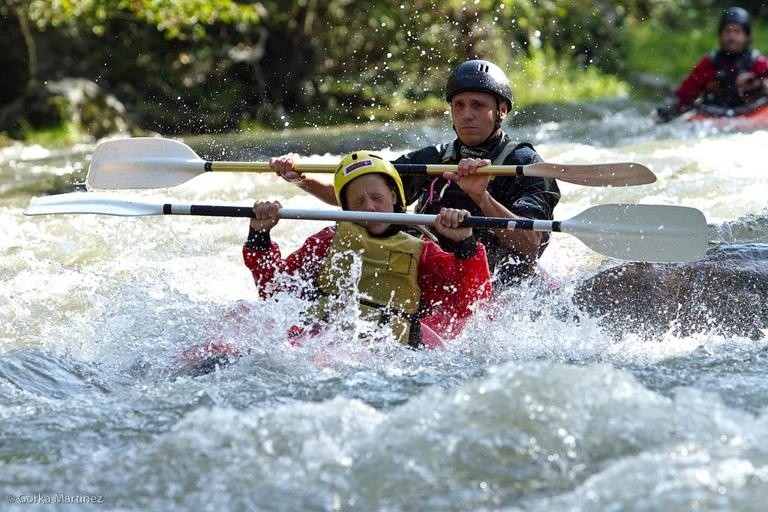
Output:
[268,59,562,298]
[649,6,768,125]
[163,150,495,348]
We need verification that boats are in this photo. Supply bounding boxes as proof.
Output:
[658,98,768,137]
[178,261,563,373]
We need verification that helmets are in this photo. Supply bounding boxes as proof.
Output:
[719,7,751,36]
[334,151,407,212]
[446,60,512,114]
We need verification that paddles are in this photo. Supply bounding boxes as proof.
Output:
[86,138,656,190]
[24,191,707,263]
